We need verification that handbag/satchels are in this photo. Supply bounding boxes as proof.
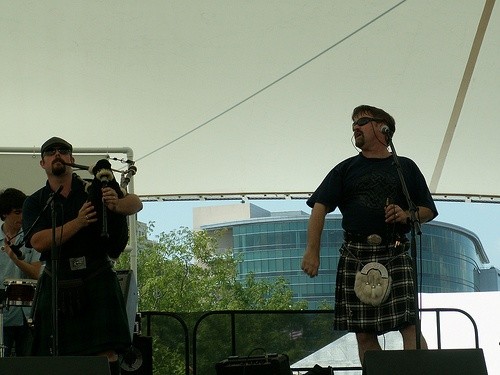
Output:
[353,262,393,305]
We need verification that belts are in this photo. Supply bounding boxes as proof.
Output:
[45,250,109,273]
[342,232,407,246]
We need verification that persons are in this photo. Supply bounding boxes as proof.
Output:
[21,136,142,375]
[301,104,438,368]
[0,187,45,359]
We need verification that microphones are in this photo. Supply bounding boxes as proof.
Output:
[7,240,25,260]
[379,123,390,133]
[122,165,137,188]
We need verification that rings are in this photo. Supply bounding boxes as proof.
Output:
[86,215,89,219]
[395,213,398,217]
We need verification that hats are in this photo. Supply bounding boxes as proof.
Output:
[41,137,73,155]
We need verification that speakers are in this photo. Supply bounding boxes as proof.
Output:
[0,356,111,375]
[363,348,487,375]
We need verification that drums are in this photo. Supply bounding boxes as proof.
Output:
[3,279,37,307]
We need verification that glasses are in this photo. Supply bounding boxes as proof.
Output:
[42,148,72,159]
[351,117,384,132]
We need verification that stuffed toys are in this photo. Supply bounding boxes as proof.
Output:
[85,158,129,261]
